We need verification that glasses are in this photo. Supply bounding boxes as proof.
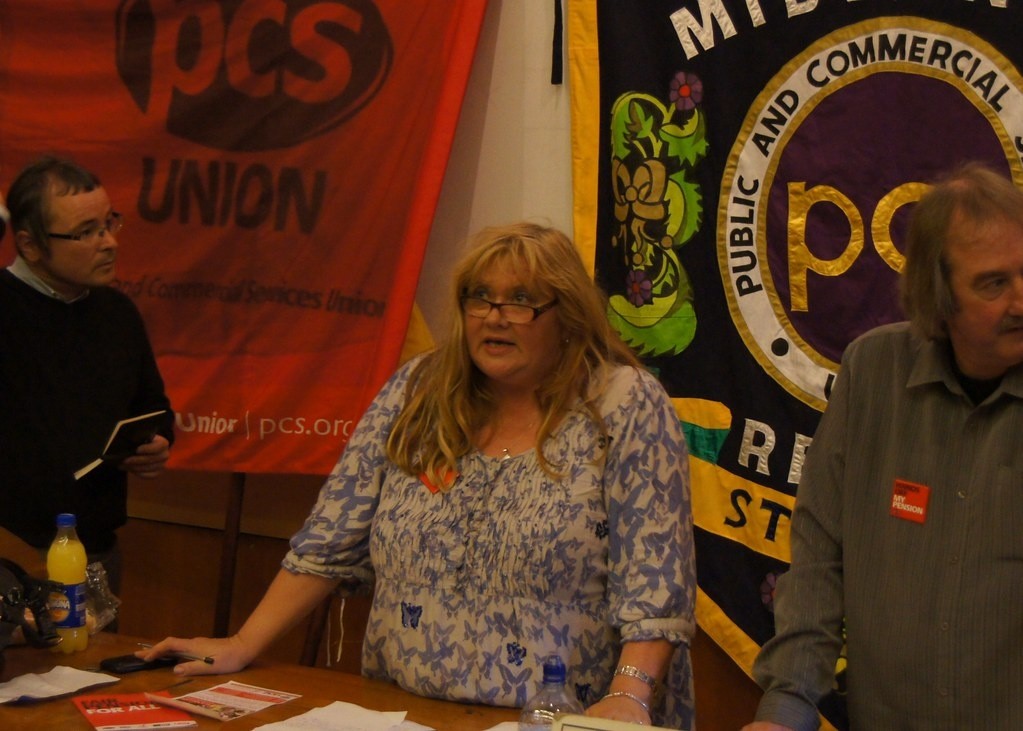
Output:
[460,294,558,324]
[32,211,123,244]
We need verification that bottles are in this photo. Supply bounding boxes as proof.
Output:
[45,510,89,654]
[516,655,583,731]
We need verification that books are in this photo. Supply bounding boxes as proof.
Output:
[70,690,199,731]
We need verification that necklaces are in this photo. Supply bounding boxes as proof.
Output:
[487,406,542,454]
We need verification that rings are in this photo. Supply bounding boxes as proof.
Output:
[639,721,644,725]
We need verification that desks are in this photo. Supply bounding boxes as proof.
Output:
[0,607,690,731]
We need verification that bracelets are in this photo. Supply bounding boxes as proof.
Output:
[599,691,650,711]
[613,665,660,698]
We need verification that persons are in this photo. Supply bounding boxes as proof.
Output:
[743,166,1023,730]
[1,154,174,641]
[134,220,698,731]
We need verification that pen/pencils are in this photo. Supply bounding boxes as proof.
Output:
[135,641,216,666]
